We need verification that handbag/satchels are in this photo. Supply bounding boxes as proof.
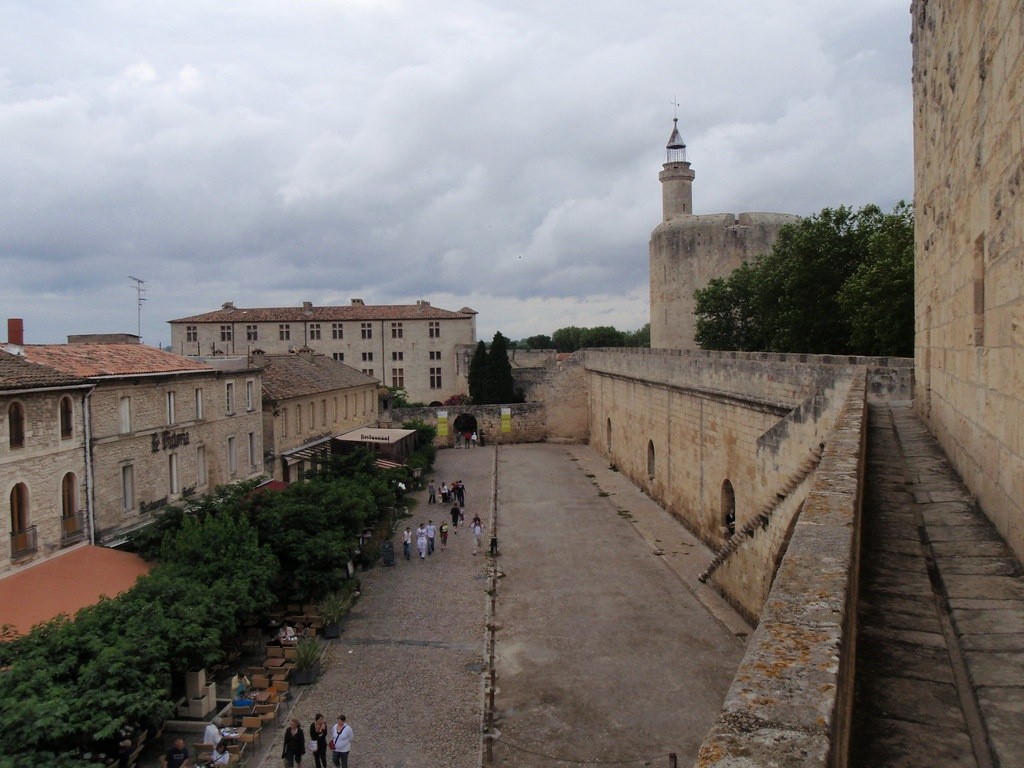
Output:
[329,740,335,750]
[309,741,317,751]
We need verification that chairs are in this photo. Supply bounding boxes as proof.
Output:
[285,605,300,627]
[251,678,269,705]
[231,705,256,727]
[282,650,298,669]
[308,628,316,636]
[249,666,269,679]
[302,606,321,620]
[193,743,224,768]
[269,666,292,682]
[226,742,247,768]
[255,703,281,732]
[292,616,308,630]
[235,717,263,756]
[309,615,323,633]
[271,681,289,709]
[267,648,282,659]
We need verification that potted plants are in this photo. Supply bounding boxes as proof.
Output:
[316,589,352,637]
[293,635,327,684]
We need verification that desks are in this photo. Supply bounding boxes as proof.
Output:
[219,727,246,753]
[263,658,286,668]
[246,692,271,704]
[267,636,303,649]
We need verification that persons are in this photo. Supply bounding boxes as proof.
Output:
[163,738,189,768]
[471,432,477,449]
[402,527,413,560]
[277,623,294,640]
[233,690,258,717]
[231,671,251,699]
[203,718,230,748]
[428,479,466,507]
[469,513,486,556]
[464,432,471,449]
[479,430,486,447]
[450,503,464,534]
[455,432,462,449]
[382,539,392,548]
[415,520,449,559]
[210,742,229,768]
[282,713,353,768]
